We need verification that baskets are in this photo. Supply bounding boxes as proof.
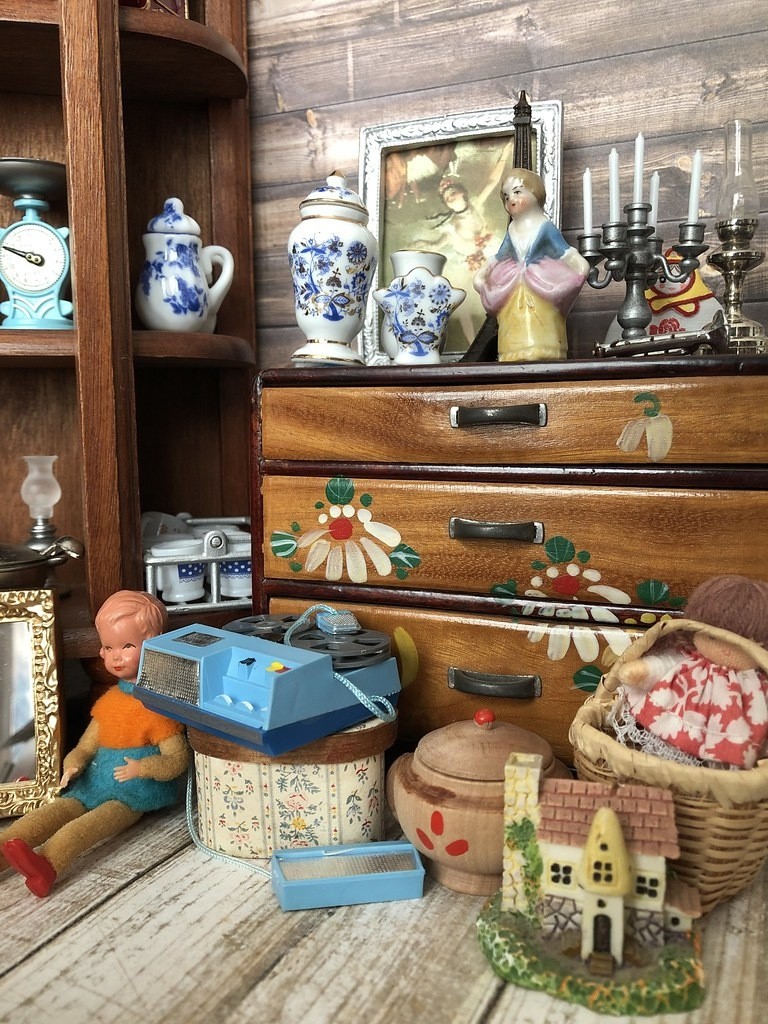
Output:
[569,615,767,918]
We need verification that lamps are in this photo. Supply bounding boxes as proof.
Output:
[19,455,70,601]
[706,119,768,357]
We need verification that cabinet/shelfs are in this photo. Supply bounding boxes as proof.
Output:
[1,0,264,657]
[252,352,768,769]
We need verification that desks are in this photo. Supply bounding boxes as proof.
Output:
[0,788,768,1023]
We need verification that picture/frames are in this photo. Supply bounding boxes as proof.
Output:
[1,590,62,817]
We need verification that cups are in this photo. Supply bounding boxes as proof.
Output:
[135,197,235,333]
[140,512,252,603]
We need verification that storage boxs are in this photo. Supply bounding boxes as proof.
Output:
[359,100,562,367]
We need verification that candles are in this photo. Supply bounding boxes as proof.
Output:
[631,132,644,204]
[647,171,660,237]
[582,167,593,235]
[688,149,702,223]
[609,147,620,223]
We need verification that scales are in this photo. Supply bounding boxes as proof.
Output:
[0,156,74,330]
